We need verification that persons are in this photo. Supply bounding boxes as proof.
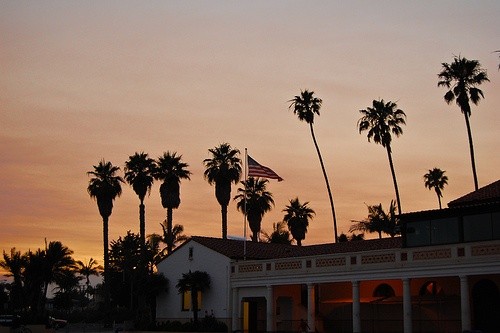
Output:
[296,318,312,333]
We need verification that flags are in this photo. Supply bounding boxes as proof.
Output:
[247,155,285,182]
